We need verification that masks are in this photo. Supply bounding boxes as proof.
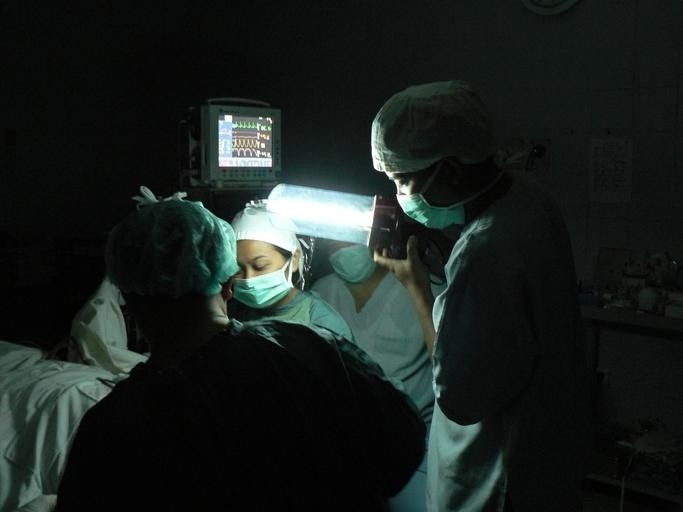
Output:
[229,260,295,311]
[394,178,468,230]
[326,244,379,285]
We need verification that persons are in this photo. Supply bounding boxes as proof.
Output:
[228,199,355,346]
[368,77,584,511]
[56,188,425,510]
[314,234,436,427]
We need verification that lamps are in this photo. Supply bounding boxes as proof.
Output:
[264,183,398,251]
